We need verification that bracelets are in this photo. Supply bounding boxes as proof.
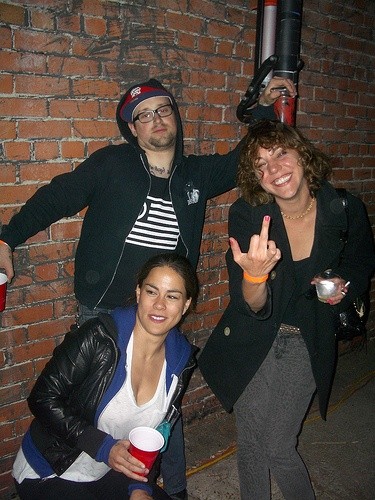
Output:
[0,240,12,250]
[243,271,268,283]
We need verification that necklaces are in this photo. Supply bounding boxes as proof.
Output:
[149,164,171,175]
[281,195,315,220]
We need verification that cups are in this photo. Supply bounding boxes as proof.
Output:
[0,273,9,313]
[128,426,165,478]
[314,271,342,304]
[270,87,295,126]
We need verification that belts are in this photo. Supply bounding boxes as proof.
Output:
[277,322,302,336]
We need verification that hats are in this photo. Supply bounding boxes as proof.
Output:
[119,85,174,123]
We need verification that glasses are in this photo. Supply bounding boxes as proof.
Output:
[132,104,173,123]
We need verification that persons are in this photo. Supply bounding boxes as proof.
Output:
[0,79,298,500]
[197,122,375,500]
[12,253,200,500]
